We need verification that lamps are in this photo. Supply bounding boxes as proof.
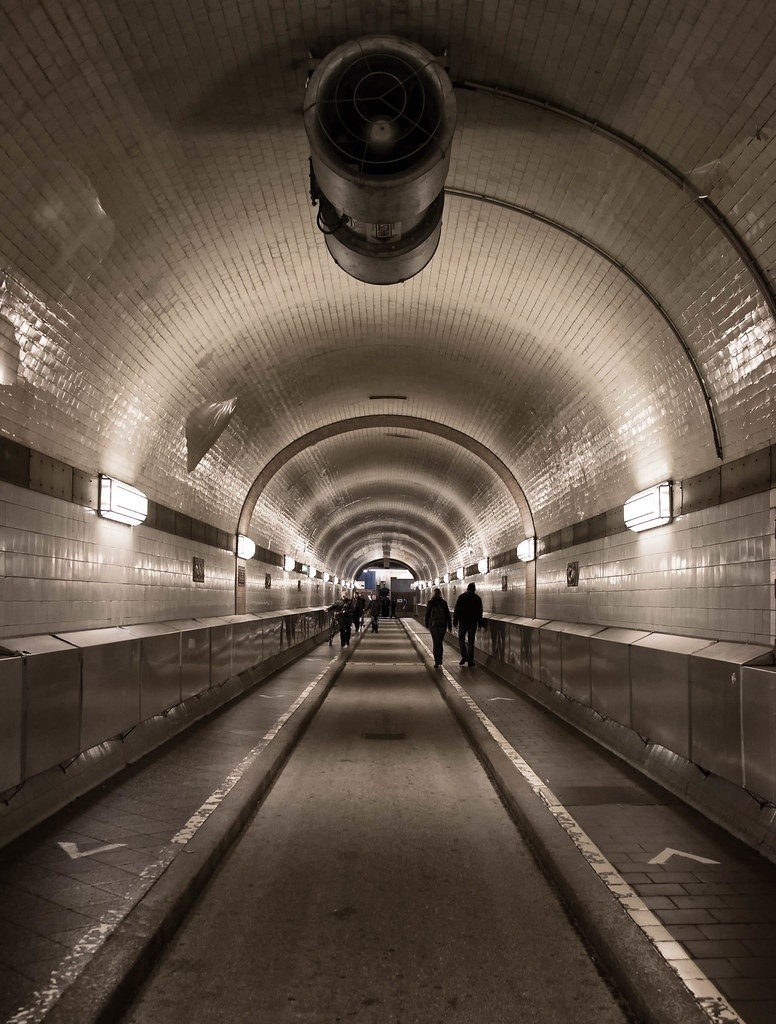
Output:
[237,532,256,560]
[341,579,354,590]
[443,573,450,584]
[321,572,329,582]
[419,583,426,590]
[434,577,440,586]
[516,537,535,563]
[478,557,489,574]
[97,473,149,527]
[456,567,465,580]
[284,555,295,572]
[332,575,339,585]
[428,581,433,588]
[623,482,672,532]
[308,565,316,578]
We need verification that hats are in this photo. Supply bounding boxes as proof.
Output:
[467,583,476,591]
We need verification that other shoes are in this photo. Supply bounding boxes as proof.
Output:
[340,640,350,647]
[438,660,443,665]
[466,661,475,667]
[460,658,468,665]
[390,616,397,619]
[355,627,359,632]
[434,663,439,668]
[371,627,378,634]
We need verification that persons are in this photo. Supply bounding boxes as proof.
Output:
[425,588,452,668]
[381,598,398,618]
[453,582,483,667]
[324,592,380,649]
